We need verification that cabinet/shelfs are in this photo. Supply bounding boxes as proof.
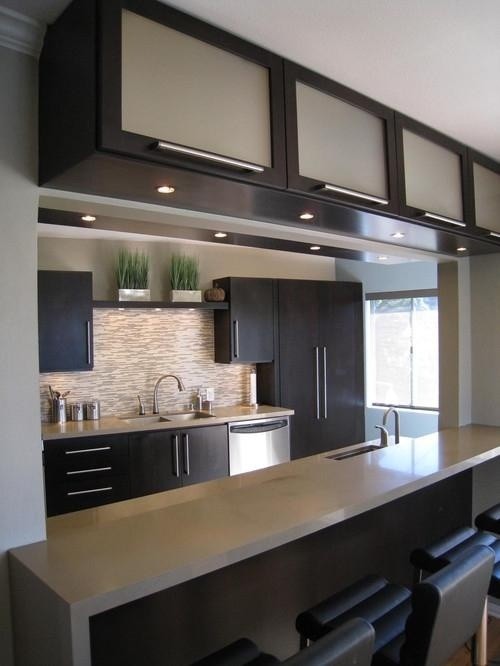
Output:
[257,277,365,462]
[212,274,273,364]
[43,424,230,518]
[36,270,94,374]
[37,0,500,258]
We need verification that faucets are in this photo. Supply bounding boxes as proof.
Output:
[382,408,400,444]
[325,445,383,461]
[153,375,186,413]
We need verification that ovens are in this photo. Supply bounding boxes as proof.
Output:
[227,416,294,478]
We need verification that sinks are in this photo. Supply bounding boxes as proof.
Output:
[118,415,172,427]
[164,409,215,423]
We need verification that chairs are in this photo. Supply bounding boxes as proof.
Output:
[186,618,376,666]
[410,525,500,601]
[294,544,496,666]
